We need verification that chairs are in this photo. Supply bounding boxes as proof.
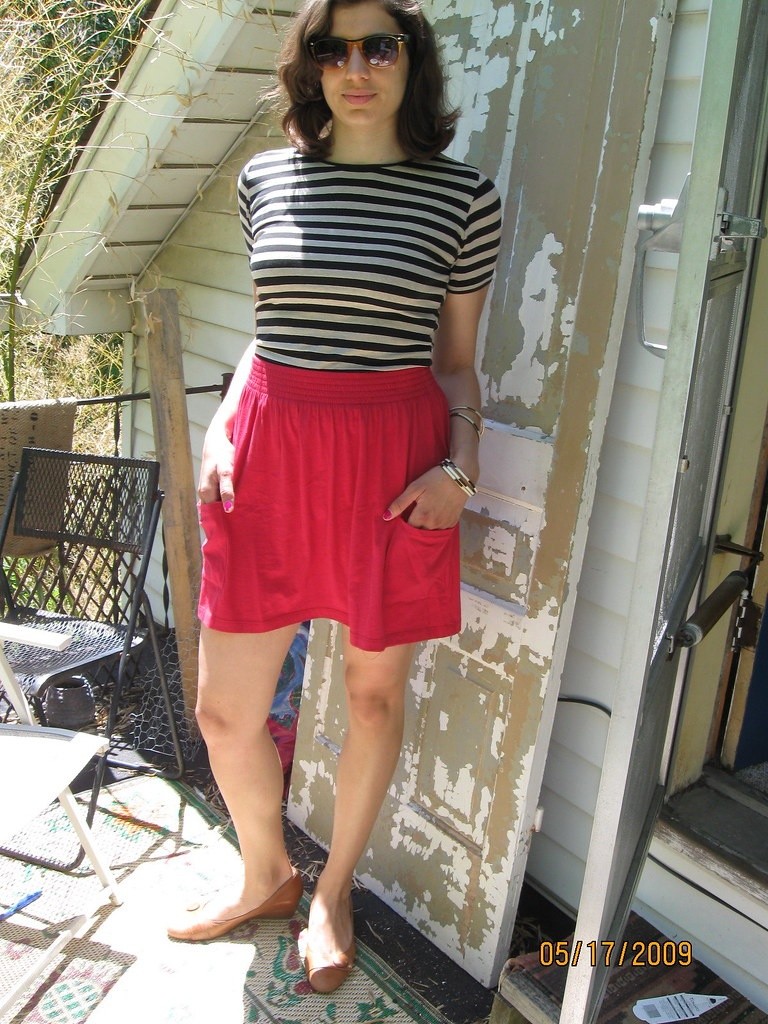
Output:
[1,621,126,1024]
[2,445,189,876]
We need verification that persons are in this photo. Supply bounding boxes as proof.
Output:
[166,3,502,996]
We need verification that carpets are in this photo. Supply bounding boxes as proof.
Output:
[2,771,455,1024]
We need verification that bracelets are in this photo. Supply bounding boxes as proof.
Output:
[439,459,477,498]
[449,407,484,444]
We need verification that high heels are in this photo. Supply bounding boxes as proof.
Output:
[167,866,302,940]
[305,867,356,992]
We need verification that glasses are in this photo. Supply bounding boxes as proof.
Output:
[309,33,410,72]
